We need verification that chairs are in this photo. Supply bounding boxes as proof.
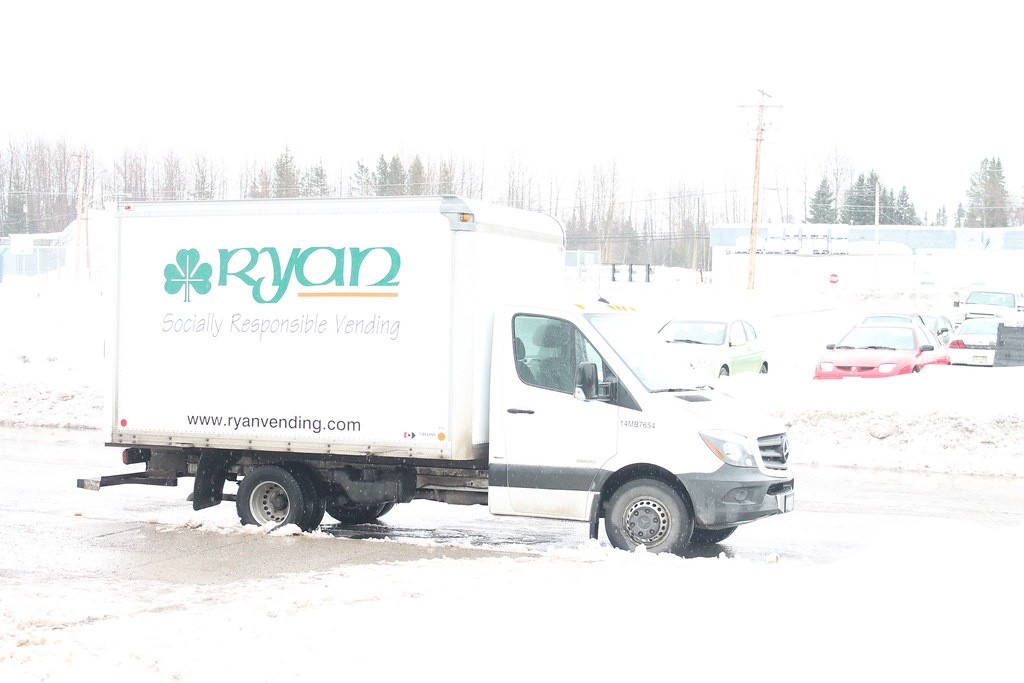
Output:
[534,324,573,391]
[515,337,536,384]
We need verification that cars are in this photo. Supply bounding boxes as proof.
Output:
[656,316,770,381]
[811,290,1024,380]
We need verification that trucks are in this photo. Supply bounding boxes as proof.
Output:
[73,193,804,559]
